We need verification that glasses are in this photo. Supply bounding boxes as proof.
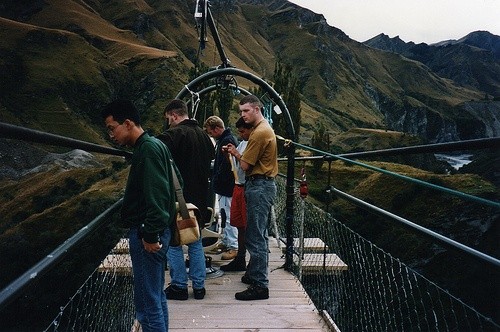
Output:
[107,124,120,135]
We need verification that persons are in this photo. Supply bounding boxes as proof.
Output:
[153,99,216,301]
[184,104,254,281]
[101,99,184,332]
[234,94,278,301]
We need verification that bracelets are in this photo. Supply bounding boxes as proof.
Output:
[240,155,243,158]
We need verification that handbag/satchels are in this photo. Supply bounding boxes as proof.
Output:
[176,202,200,245]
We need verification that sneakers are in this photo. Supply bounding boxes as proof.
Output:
[242,276,255,284]
[193,287,205,299]
[164,285,188,300]
[235,281,269,300]
[221,250,237,260]
[220,256,247,271]
